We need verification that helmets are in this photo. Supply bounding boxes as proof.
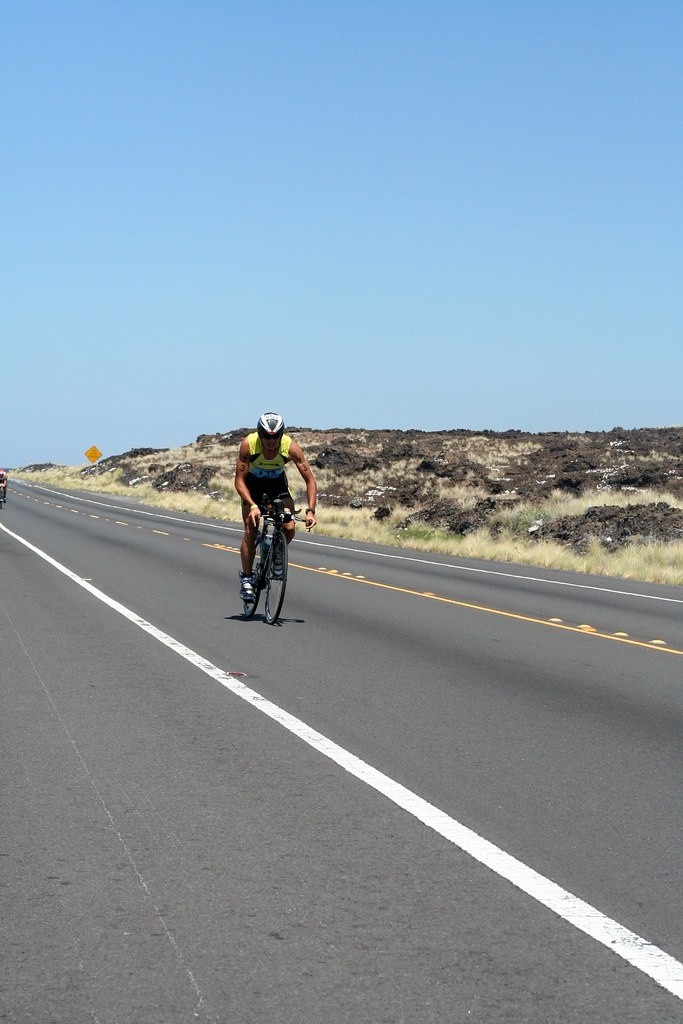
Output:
[0,468,4,474]
[257,412,284,435]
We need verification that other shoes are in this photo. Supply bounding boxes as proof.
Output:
[3,496,7,503]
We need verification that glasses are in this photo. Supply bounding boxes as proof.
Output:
[261,433,281,440]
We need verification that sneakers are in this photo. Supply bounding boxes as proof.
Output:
[274,552,283,577]
[239,577,253,599]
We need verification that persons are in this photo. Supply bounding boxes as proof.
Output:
[235,411,318,600]
[0,468,7,503]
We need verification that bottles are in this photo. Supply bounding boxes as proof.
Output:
[262,534,273,560]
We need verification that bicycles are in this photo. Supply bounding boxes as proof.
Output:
[0,483,6,509]
[240,490,312,626]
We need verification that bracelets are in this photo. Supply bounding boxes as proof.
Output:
[250,505,258,510]
[306,508,315,514]
